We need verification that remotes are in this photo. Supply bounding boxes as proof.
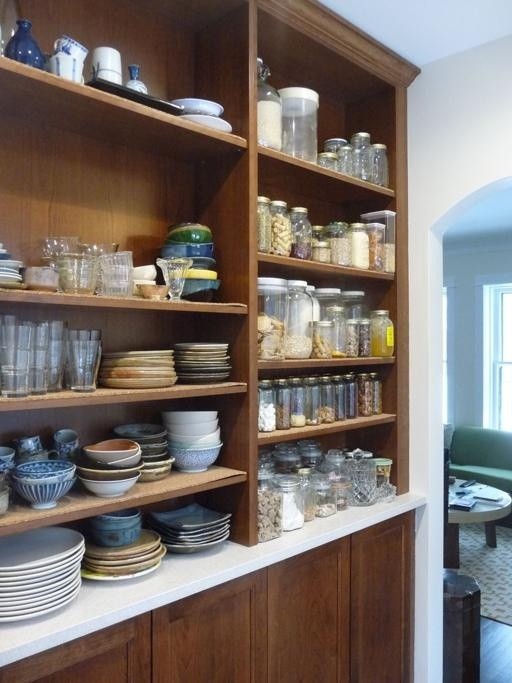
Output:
[459,479,476,488]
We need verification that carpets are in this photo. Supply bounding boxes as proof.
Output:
[443,521,512,625]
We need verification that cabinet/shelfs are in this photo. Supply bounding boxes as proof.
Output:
[1,1,421,682]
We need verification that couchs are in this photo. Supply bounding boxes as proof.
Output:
[444,423,512,527]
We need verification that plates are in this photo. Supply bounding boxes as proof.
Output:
[180,115,235,134]
[0,241,26,291]
[0,504,231,624]
[171,98,225,116]
[102,341,233,388]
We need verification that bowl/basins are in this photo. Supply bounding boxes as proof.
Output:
[92,509,143,547]
[0,411,223,515]
[134,222,221,302]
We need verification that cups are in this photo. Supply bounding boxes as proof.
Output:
[44,236,135,297]
[0,314,101,399]
[48,34,122,85]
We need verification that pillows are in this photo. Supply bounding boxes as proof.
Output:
[444,424,455,461]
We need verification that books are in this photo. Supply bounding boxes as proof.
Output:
[450,496,477,512]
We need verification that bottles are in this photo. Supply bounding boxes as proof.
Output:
[3,20,43,70]
[257,373,385,433]
[127,65,147,95]
[257,195,396,274]
[257,440,396,543]
[258,57,390,188]
[259,278,396,361]
